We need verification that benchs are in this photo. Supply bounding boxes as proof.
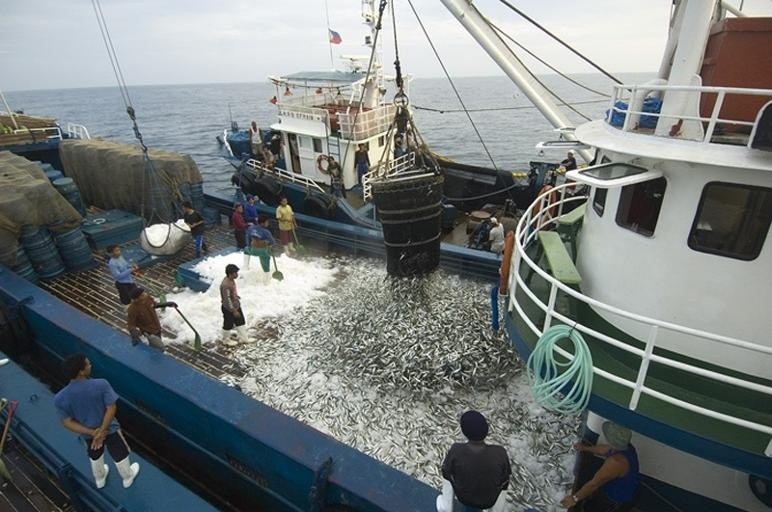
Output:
[524,231,582,311]
[555,202,586,265]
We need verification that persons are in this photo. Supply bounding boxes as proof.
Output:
[107,245,138,305]
[276,197,296,256]
[182,201,209,258]
[54,353,140,488]
[556,150,577,196]
[127,288,177,352]
[232,203,253,251]
[249,87,403,198]
[436,411,512,512]
[480,217,505,254]
[244,216,276,272]
[220,264,257,346]
[560,422,639,512]
[243,195,259,225]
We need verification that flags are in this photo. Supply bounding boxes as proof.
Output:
[329,29,342,44]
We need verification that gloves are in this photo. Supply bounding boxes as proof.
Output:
[156,301,178,307]
[131,331,139,346]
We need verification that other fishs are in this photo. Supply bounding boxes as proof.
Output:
[205,250,582,512]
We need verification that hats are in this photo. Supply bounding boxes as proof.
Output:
[603,422,632,450]
[460,411,489,442]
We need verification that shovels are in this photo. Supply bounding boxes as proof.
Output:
[291,221,305,255]
[271,248,283,281]
[134,271,166,311]
[174,306,201,351]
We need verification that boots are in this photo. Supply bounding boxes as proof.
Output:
[242,254,250,271]
[90,454,109,489]
[488,491,509,512]
[235,324,257,343]
[222,325,238,346]
[264,271,272,286]
[436,479,453,512]
[123,304,130,316]
[114,455,139,488]
[283,241,295,255]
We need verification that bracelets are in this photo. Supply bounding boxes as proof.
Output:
[572,496,578,502]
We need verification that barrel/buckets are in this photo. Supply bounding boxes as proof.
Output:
[0,150,96,284]
